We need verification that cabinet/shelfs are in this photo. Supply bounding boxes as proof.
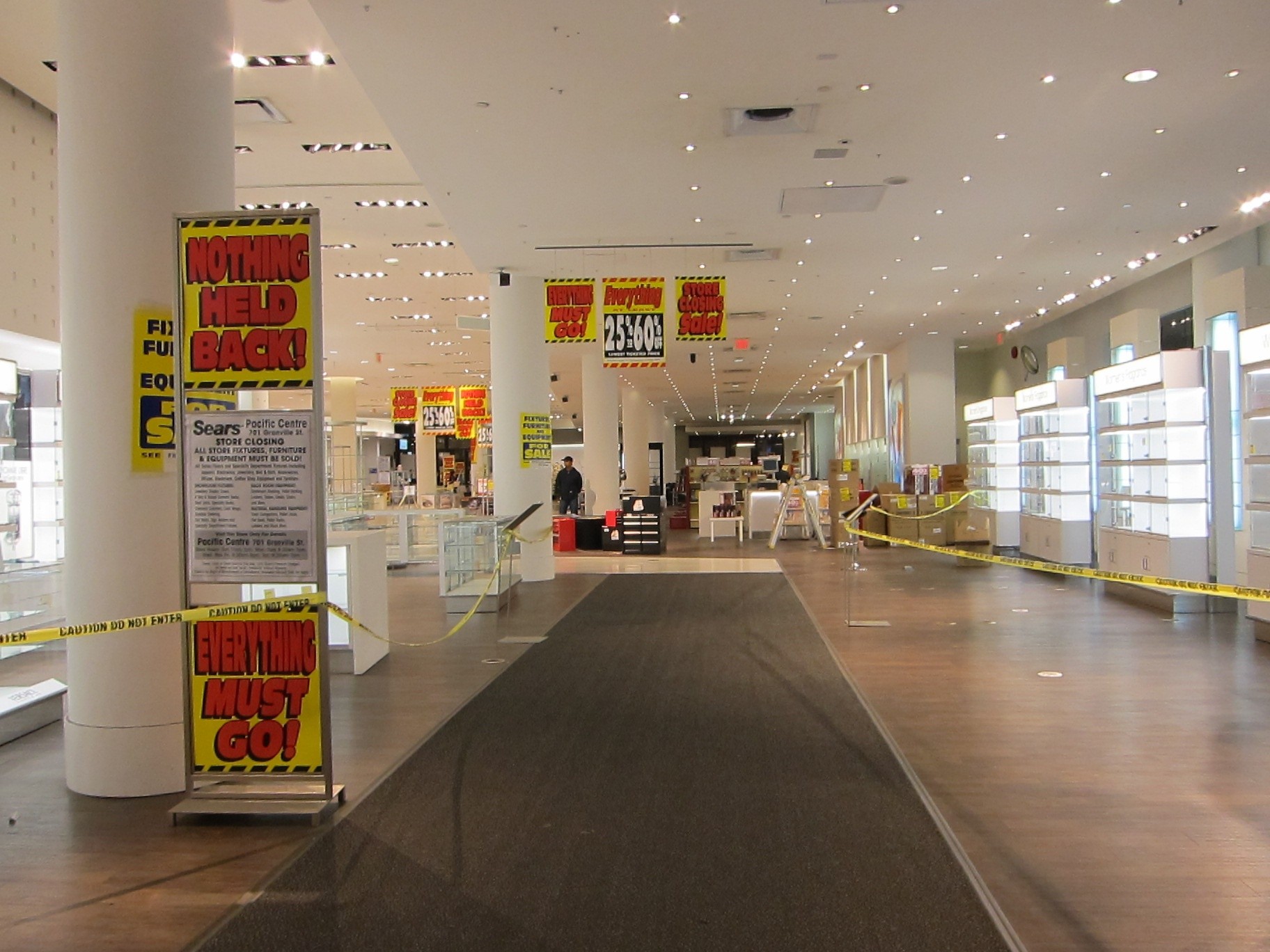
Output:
[324,420,369,531]
[369,515,463,564]
[0,393,70,746]
[1091,347,1209,613]
[685,465,764,528]
[962,396,1021,549]
[239,533,386,675]
[1237,321,1270,624]
[1015,377,1095,566]
[780,490,832,539]
[551,517,575,550]
[439,514,524,614]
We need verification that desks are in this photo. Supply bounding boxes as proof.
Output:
[710,516,745,543]
[698,490,738,537]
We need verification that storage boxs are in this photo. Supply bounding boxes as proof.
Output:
[828,459,992,566]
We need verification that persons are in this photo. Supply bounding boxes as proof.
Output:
[555,456,582,515]
[449,469,458,493]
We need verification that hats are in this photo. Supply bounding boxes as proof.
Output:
[561,456,572,461]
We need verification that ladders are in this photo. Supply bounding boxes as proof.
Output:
[767,473,827,549]
